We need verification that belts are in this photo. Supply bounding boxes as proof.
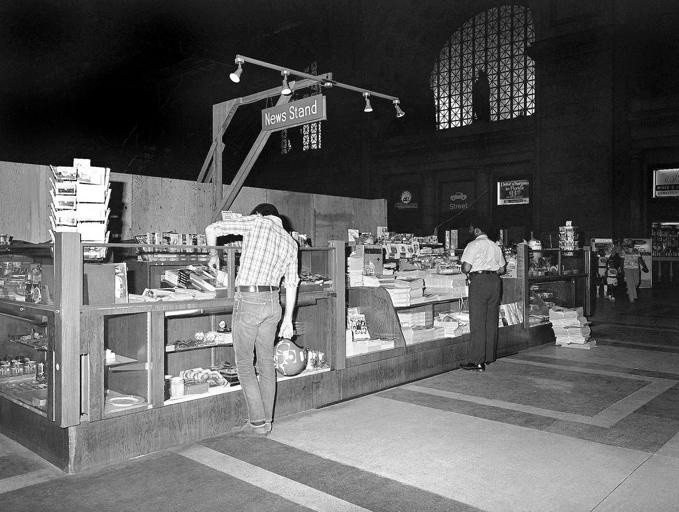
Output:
[234,286,279,293]
[469,271,497,275]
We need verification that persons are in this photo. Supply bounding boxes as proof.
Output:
[596,233,649,304]
[460,221,507,373]
[203,204,301,437]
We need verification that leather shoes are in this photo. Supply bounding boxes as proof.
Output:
[460,358,496,373]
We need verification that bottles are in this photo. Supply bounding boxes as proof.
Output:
[3,261,13,278]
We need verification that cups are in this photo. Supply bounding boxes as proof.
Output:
[164,376,186,401]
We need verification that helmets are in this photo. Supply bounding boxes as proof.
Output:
[274,338,308,376]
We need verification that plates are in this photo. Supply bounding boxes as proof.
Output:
[104,394,147,407]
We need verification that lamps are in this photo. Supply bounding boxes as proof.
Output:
[228,54,406,119]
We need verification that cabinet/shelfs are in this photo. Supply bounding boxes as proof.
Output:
[0,232,592,474]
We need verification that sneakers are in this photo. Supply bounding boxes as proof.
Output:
[232,422,271,436]
